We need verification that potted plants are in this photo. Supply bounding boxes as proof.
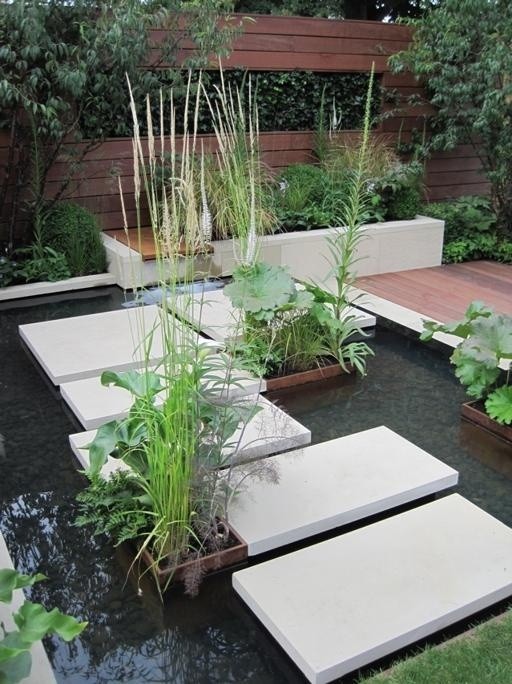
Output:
[420,300,512,443]
[73,70,284,587]
[223,61,375,391]
[145,68,444,281]
